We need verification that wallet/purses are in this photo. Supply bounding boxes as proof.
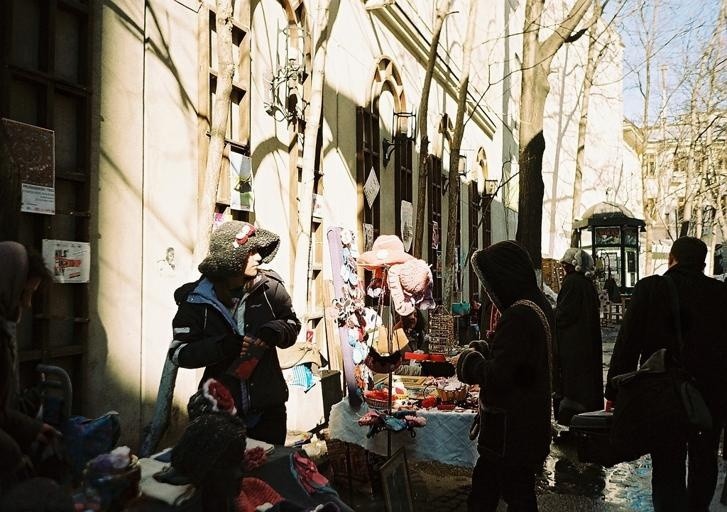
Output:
[225,333,267,381]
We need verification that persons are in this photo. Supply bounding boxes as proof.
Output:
[171,412,306,512]
[552,248,604,426]
[169,220,302,446]
[0,240,64,446]
[452,240,555,512]
[605,236,727,512]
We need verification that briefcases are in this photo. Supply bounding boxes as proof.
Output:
[568,409,623,469]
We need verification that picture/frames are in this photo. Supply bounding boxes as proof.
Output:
[383,374,426,386]
[378,447,415,512]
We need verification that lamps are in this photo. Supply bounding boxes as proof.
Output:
[383,108,417,168]
[441,154,467,195]
[469,179,498,210]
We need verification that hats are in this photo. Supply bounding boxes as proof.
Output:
[356,234,436,317]
[670,236,706,266]
[560,247,597,279]
[199,219,281,280]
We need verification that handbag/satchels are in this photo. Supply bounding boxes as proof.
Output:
[378,325,410,353]
[608,346,712,453]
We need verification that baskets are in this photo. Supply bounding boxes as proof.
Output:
[435,385,467,402]
[362,395,396,410]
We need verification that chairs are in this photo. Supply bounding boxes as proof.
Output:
[610,287,635,323]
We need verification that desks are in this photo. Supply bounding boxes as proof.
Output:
[339,345,479,510]
